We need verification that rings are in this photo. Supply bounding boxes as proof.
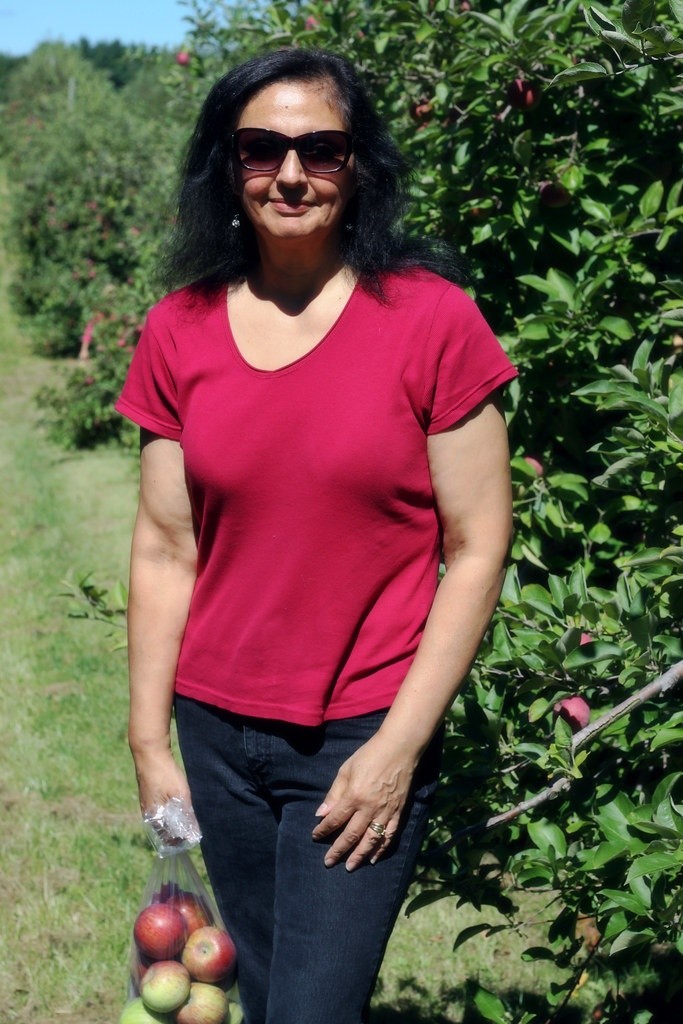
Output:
[369,823,387,837]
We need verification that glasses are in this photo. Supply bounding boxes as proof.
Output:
[228,126,360,174]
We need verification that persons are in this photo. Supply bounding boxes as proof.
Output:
[113,54,520,1024]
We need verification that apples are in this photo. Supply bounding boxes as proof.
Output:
[47,0,683,1024]
